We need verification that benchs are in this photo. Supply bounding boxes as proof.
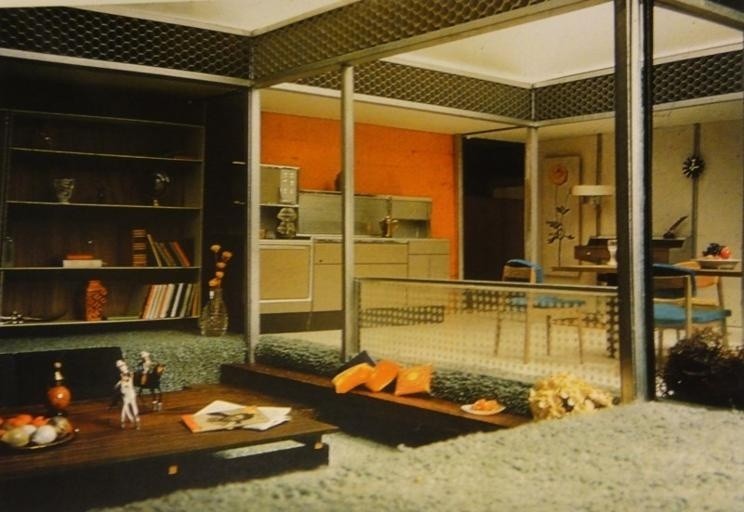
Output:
[221,358,533,441]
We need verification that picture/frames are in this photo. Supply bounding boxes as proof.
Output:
[539,152,585,280]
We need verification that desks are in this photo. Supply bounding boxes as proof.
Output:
[553,265,742,359]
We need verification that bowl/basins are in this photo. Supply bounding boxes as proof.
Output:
[693,258,740,269]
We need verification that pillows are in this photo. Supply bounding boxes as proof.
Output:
[332,351,437,402]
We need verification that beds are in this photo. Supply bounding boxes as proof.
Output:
[675,260,733,348]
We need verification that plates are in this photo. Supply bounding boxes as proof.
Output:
[0,426,79,450]
[460,403,505,415]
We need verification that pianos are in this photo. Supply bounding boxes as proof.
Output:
[573,235,683,284]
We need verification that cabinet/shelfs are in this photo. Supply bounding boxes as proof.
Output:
[0,106,206,327]
[261,164,450,330]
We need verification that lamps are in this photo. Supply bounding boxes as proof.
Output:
[570,184,615,236]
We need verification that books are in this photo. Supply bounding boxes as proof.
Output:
[61,226,192,268]
[129,280,200,320]
[182,399,293,434]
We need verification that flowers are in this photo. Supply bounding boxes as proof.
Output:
[544,163,575,270]
[208,243,234,291]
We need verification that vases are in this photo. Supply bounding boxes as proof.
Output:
[200,287,229,337]
[78,280,108,322]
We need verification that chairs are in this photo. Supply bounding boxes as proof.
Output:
[492,259,586,365]
[652,263,731,369]
